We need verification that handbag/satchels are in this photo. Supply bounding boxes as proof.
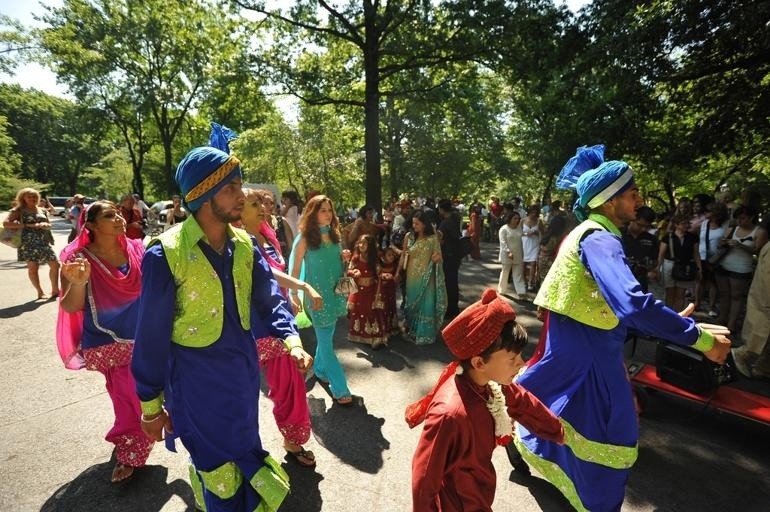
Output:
[371,299,385,311]
[0,207,24,248]
[671,259,697,283]
[333,271,360,296]
[163,209,177,232]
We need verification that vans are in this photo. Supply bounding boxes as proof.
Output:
[48,197,99,218]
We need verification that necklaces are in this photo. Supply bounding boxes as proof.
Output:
[466,380,518,447]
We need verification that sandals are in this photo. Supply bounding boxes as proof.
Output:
[335,395,353,406]
[110,462,136,485]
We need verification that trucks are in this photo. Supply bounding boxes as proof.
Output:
[156,183,283,234]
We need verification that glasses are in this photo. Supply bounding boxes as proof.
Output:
[634,220,651,229]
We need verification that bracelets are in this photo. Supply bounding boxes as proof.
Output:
[141,414,166,424]
[24,222,28,232]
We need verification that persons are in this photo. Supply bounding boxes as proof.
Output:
[41,190,50,208]
[39,197,56,213]
[237,190,319,469]
[2,186,60,303]
[51,200,167,482]
[505,138,734,512]
[401,287,570,511]
[128,121,316,511]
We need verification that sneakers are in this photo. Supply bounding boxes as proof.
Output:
[708,309,718,318]
[731,343,770,382]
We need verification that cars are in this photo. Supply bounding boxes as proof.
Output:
[146,200,177,221]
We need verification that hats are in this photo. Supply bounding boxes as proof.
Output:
[574,159,637,212]
[439,286,518,362]
[172,144,246,213]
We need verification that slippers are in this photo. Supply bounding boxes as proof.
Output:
[282,443,317,471]
